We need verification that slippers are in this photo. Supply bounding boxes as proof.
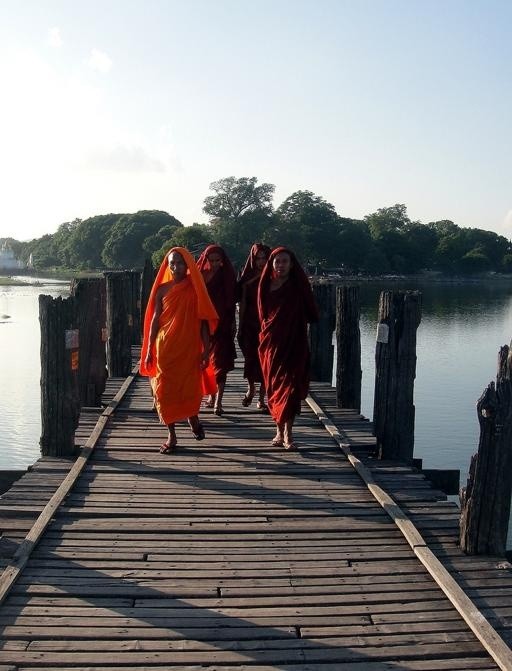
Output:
[205,398,215,408]
[213,405,225,418]
[283,439,297,451]
[188,416,206,441]
[256,401,267,412]
[271,435,284,447]
[159,438,179,455]
[241,394,253,408]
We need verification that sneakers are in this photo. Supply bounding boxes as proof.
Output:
[289,446,291,448]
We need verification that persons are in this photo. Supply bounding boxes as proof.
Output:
[254,247,316,454]
[195,245,237,417]
[234,243,274,412]
[139,246,224,457]
